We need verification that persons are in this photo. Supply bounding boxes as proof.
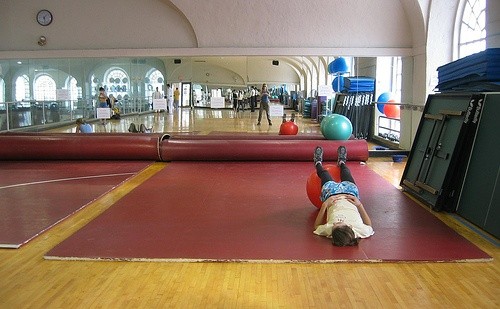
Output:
[193,89,211,105]
[282,112,295,123]
[128,122,154,133]
[250,86,260,112]
[174,87,180,111]
[166,84,173,114]
[98,87,108,124]
[75,117,93,133]
[232,89,250,113]
[153,87,162,113]
[313,145,375,246]
[257,83,273,126]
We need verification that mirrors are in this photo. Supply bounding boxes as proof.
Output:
[0,56,402,150]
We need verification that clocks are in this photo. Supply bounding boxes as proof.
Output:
[36,9,53,26]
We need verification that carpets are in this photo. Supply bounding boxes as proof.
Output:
[0,136,493,264]
[0,131,323,140]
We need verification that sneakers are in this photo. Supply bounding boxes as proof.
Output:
[313,146,324,166]
[338,146,348,165]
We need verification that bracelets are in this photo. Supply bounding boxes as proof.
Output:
[357,202,363,209]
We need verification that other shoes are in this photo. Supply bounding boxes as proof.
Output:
[102,122,106,125]
[257,121,260,125]
[269,121,272,125]
